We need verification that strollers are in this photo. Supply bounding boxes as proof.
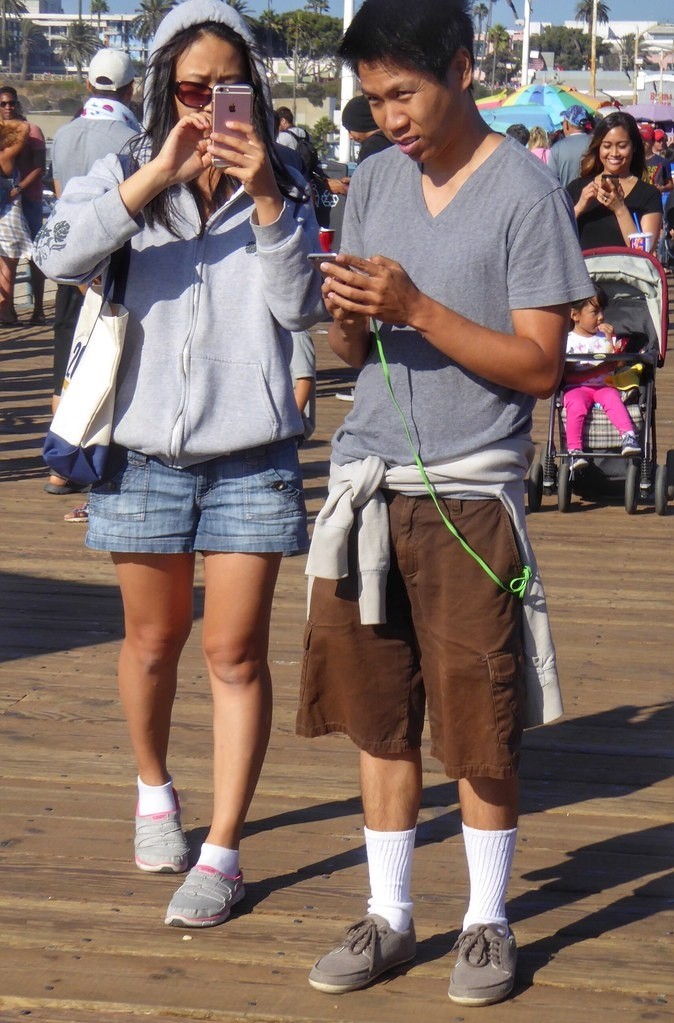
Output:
[529,247,674,516]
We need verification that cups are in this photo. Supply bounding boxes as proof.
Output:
[628,233,653,253]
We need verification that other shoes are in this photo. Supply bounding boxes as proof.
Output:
[0,317,23,326]
[64,499,91,522]
[29,313,45,325]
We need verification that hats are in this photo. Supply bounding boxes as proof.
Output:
[341,96,379,133]
[560,105,589,127]
[89,48,135,92]
[637,127,655,140]
[653,129,665,141]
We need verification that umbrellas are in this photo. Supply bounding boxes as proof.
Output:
[619,102,674,122]
[499,79,601,125]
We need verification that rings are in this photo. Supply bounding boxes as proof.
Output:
[605,197,607,200]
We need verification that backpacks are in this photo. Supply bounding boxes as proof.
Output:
[285,130,317,176]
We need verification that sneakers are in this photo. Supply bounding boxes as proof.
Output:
[134,785,188,873]
[309,914,416,993]
[568,448,588,481]
[448,925,517,1007]
[165,867,245,927]
[621,434,641,456]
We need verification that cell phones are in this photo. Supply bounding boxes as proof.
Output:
[601,173,619,193]
[313,172,325,182]
[211,84,256,168]
[307,253,364,289]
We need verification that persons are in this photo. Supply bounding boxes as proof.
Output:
[323,96,394,402]
[565,283,641,470]
[30,0,329,926]
[548,105,593,188]
[44,48,140,494]
[506,124,551,165]
[273,107,313,184]
[637,123,674,273]
[0,86,45,327]
[565,112,664,255]
[296,0,596,1009]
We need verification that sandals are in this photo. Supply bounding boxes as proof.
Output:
[45,472,92,495]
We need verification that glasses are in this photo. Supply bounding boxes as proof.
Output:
[175,81,213,108]
[0,101,16,108]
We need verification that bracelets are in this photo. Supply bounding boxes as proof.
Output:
[15,186,22,192]
